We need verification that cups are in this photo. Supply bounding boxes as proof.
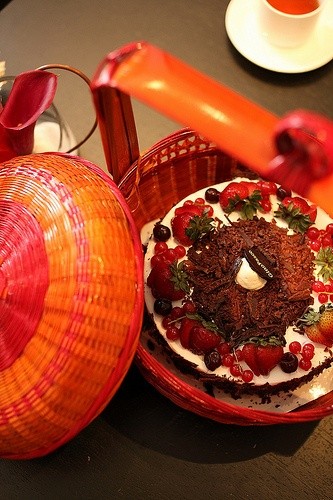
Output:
[257,0,322,48]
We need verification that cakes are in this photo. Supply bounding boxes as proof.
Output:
[141,178,333,398]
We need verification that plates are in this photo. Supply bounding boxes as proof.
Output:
[224,0,333,73]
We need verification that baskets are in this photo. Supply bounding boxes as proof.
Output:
[89,40,333,424]
